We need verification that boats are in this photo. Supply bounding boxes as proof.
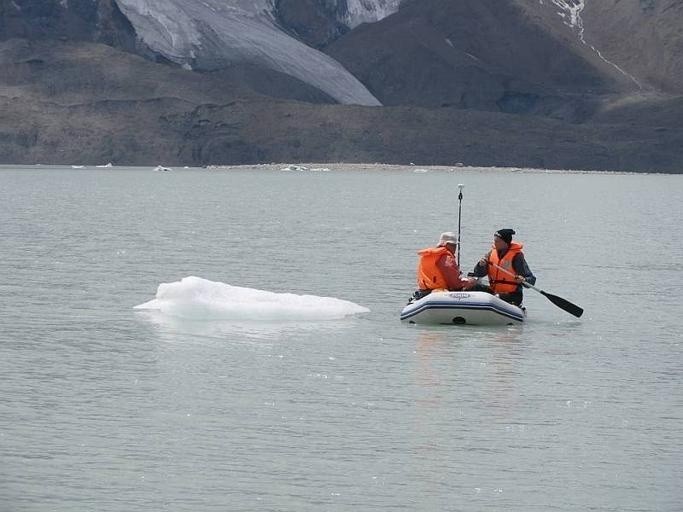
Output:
[399,182,526,327]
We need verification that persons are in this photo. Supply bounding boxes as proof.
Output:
[417,233,478,294]
[474,229,536,306]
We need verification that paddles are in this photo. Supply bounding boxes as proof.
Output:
[484,259,584,317]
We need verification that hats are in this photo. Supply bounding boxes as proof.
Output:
[495,229,515,243]
[437,231,459,247]
